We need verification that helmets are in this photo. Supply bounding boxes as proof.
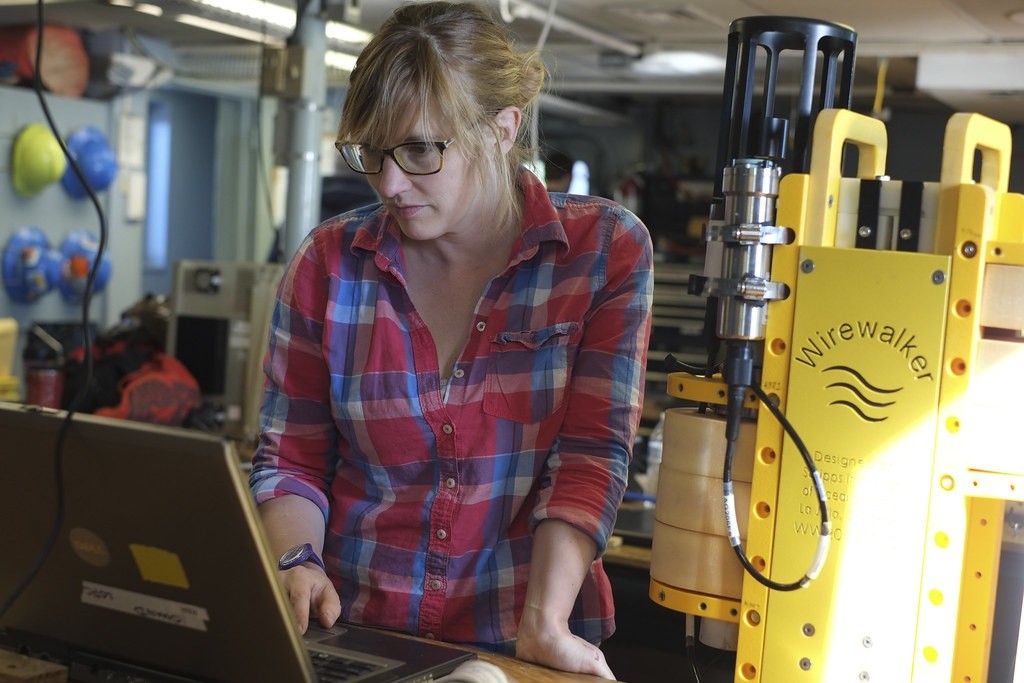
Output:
[60,125,118,200]
[58,229,113,306]
[2,226,65,305]
[11,124,66,198]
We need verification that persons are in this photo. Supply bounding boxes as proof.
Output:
[248,2,654,680]
[544,150,573,193]
[611,132,706,264]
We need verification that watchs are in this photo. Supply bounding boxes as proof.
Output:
[278,543,323,569]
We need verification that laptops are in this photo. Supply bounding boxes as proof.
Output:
[0,401,477,683]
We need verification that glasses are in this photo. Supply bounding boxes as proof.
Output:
[335,108,503,176]
